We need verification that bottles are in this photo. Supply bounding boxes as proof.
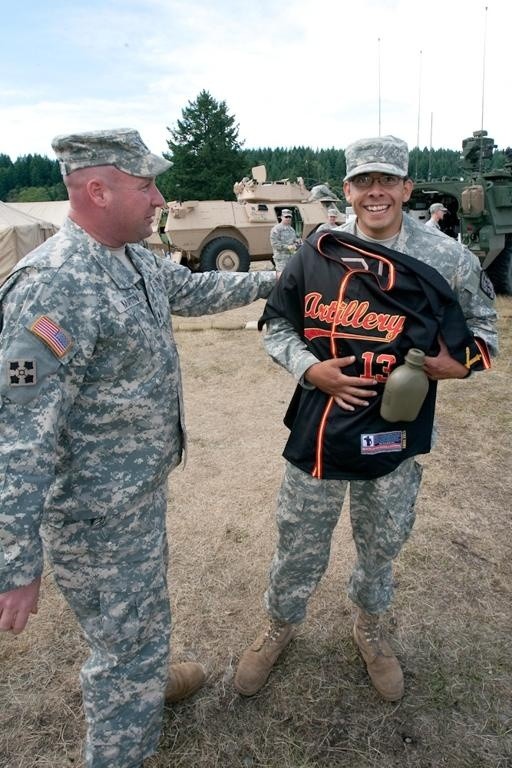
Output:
[379,349,430,424]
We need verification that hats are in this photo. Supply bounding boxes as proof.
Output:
[281,209,292,217]
[51,128,174,177]
[343,135,409,182]
[327,209,339,217]
[429,203,448,213]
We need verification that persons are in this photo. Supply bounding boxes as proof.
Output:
[0,129,285,768]
[270,207,302,269]
[234,136,501,702]
[425,202,449,229]
[311,183,335,198]
[316,208,338,235]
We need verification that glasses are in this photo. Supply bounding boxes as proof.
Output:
[350,175,404,187]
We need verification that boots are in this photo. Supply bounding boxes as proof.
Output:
[234,617,293,696]
[352,608,405,702]
[167,662,205,703]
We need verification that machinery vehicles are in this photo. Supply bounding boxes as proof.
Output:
[402,130,512,296]
[143,165,346,272]
